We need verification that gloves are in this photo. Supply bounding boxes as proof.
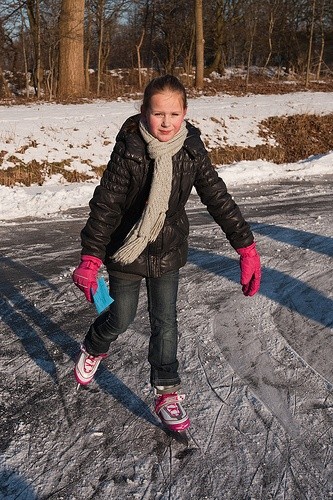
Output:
[236,241,260,296]
[72,255,103,303]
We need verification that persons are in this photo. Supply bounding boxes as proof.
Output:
[72,75,261,446]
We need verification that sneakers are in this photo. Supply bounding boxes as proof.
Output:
[153,384,190,430]
[73,345,108,385]
